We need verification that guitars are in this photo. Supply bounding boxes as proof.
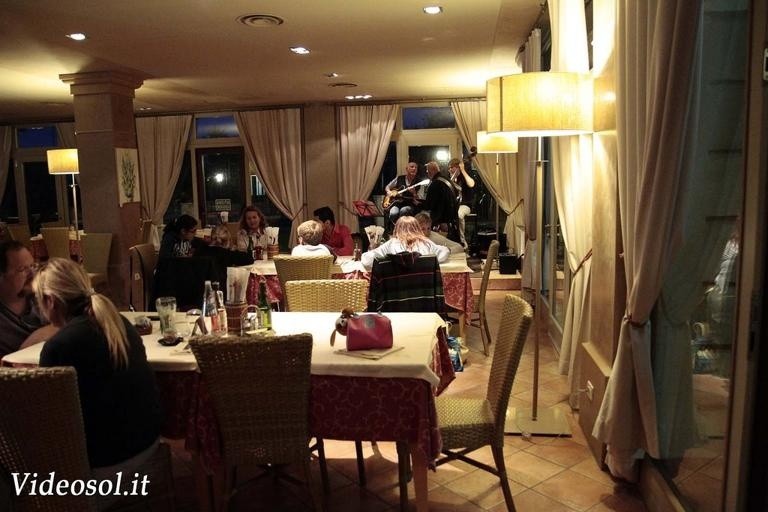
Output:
[382,178,431,213]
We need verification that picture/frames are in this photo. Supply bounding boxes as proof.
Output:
[116,146,141,206]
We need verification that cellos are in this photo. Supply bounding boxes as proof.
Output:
[450,146,477,185]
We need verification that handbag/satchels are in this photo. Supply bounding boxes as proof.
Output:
[344,312,395,351]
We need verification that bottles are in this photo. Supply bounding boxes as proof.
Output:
[258,282,272,330]
[247,236,255,263]
[215,291,230,337]
[203,282,219,337]
[212,282,220,308]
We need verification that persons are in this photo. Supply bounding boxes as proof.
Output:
[1,240,60,366]
[361,216,450,272]
[156,214,206,306]
[414,161,459,242]
[385,162,424,240]
[31,257,161,489]
[291,220,337,265]
[414,211,463,253]
[311,206,354,256]
[447,158,482,253]
[187,225,254,311]
[235,205,276,261]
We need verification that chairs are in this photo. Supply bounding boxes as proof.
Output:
[285,279,380,487]
[39,227,70,261]
[223,223,241,247]
[77,233,117,307]
[0,368,174,510]
[431,195,460,238]
[8,223,31,247]
[457,193,486,231]
[129,242,158,313]
[275,254,334,312]
[397,294,533,510]
[368,252,446,311]
[188,332,330,511]
[450,240,501,356]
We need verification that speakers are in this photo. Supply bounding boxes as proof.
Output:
[498,253,518,275]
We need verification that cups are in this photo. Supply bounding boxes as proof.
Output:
[255,246,264,260]
[156,296,178,335]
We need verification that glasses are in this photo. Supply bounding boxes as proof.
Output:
[9,262,39,278]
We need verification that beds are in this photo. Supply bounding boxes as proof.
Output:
[0,312,456,512]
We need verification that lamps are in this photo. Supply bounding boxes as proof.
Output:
[487,74,591,438]
[476,129,518,267]
[47,149,79,262]
